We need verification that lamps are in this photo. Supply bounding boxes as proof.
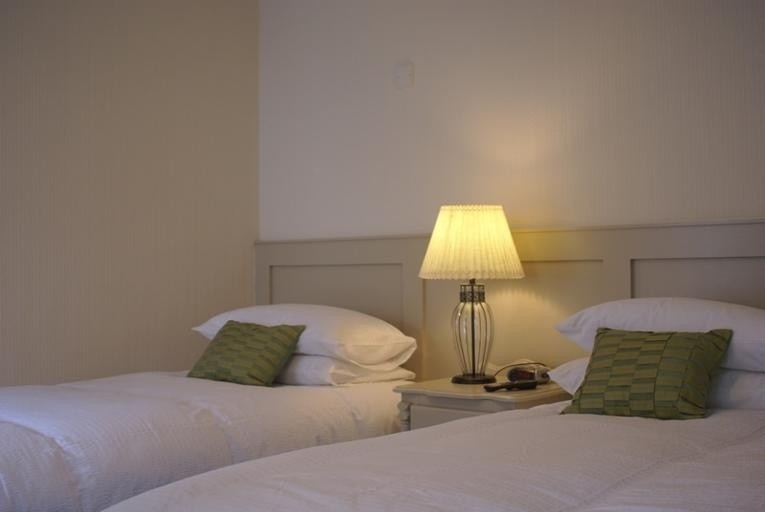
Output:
[418,204,526,385]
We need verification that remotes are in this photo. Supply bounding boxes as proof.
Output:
[487,379,536,392]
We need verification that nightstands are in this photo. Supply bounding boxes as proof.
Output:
[393,373,572,430]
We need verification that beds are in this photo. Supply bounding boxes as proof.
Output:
[103,219,765,511]
[1,236,423,511]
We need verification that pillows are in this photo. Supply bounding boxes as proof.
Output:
[274,355,416,386]
[559,326,734,420]
[191,303,417,371]
[547,357,765,411]
[553,297,765,373]
[187,320,306,387]
